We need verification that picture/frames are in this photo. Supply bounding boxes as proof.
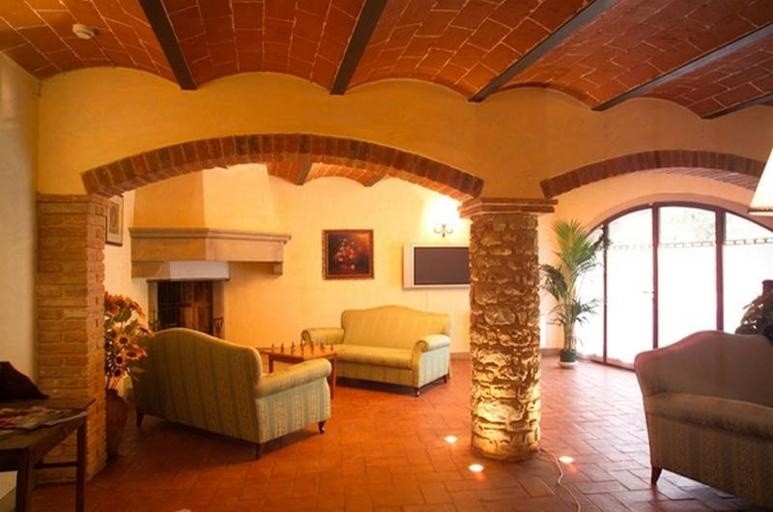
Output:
[321,228,375,280]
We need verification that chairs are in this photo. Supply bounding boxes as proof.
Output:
[634,330,772,509]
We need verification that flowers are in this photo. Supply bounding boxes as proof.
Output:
[104,291,156,390]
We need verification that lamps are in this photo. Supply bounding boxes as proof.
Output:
[433,197,456,237]
[746,147,773,219]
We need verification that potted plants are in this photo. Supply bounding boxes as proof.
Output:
[541,216,612,369]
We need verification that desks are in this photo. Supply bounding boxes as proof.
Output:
[1,397,97,512]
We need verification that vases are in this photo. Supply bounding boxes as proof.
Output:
[106,389,129,460]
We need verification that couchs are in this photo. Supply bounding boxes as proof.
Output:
[301,305,454,398]
[130,327,333,461]
